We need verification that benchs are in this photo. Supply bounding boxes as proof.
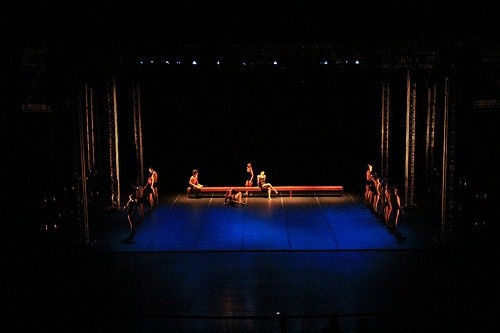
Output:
[187,185,344,198]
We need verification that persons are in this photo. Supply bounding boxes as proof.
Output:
[224,189,244,206]
[256,171,278,200]
[125,168,158,229]
[364,164,400,234]
[244,163,253,197]
[188,169,203,190]
